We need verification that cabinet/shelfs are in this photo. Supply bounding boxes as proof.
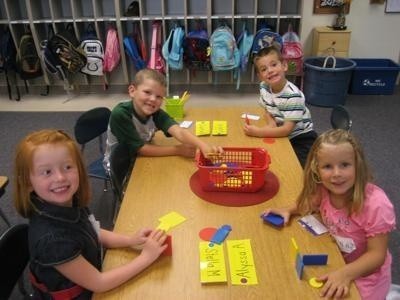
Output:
[312,26,353,58]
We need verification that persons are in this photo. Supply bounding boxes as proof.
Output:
[262,129,396,300]
[104,68,224,225]
[14,129,168,300]
[243,46,318,168]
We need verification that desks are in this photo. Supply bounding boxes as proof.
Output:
[96,104,361,300]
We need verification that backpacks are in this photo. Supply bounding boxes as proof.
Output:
[281,24,304,74]
[182,22,212,78]
[209,23,240,90]
[17,35,43,80]
[42,23,104,91]
[0,31,21,101]
[124,36,146,71]
[239,32,254,71]
[251,28,282,65]
[104,24,121,72]
[161,24,185,70]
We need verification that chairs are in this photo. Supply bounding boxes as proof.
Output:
[0,174,12,231]
[0,225,28,298]
[57,107,120,226]
[327,103,355,143]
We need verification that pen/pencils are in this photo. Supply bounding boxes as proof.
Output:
[163,97,170,104]
[179,90,190,105]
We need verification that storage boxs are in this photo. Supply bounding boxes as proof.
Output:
[193,148,272,194]
[348,58,400,95]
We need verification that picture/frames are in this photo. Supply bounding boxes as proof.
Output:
[313,1,351,14]
